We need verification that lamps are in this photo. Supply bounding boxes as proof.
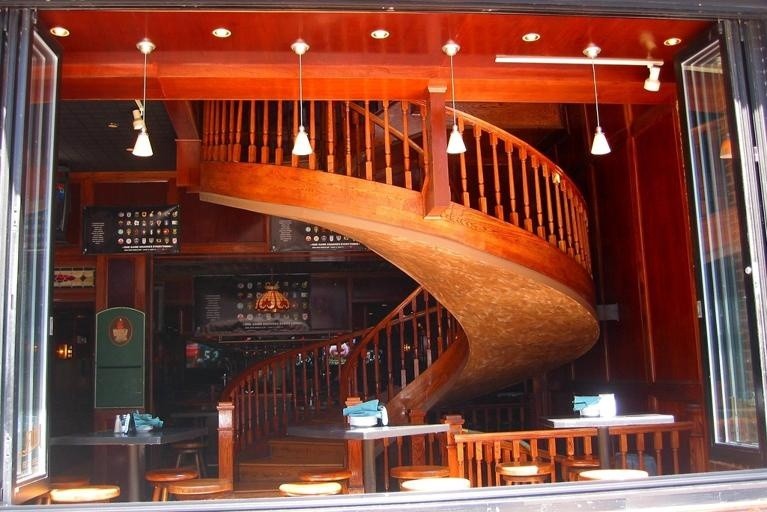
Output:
[441,44,467,155]
[132,41,155,157]
[132,99,143,130]
[254,263,291,313]
[583,47,612,155]
[644,63,660,92]
[713,56,733,159]
[291,42,313,155]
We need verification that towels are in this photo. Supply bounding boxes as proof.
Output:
[342,399,381,417]
[572,396,601,412]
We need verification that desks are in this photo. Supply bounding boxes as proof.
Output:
[48,411,218,502]
[286,423,450,494]
[538,413,674,469]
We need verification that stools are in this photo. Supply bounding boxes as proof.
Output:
[35,443,650,504]
